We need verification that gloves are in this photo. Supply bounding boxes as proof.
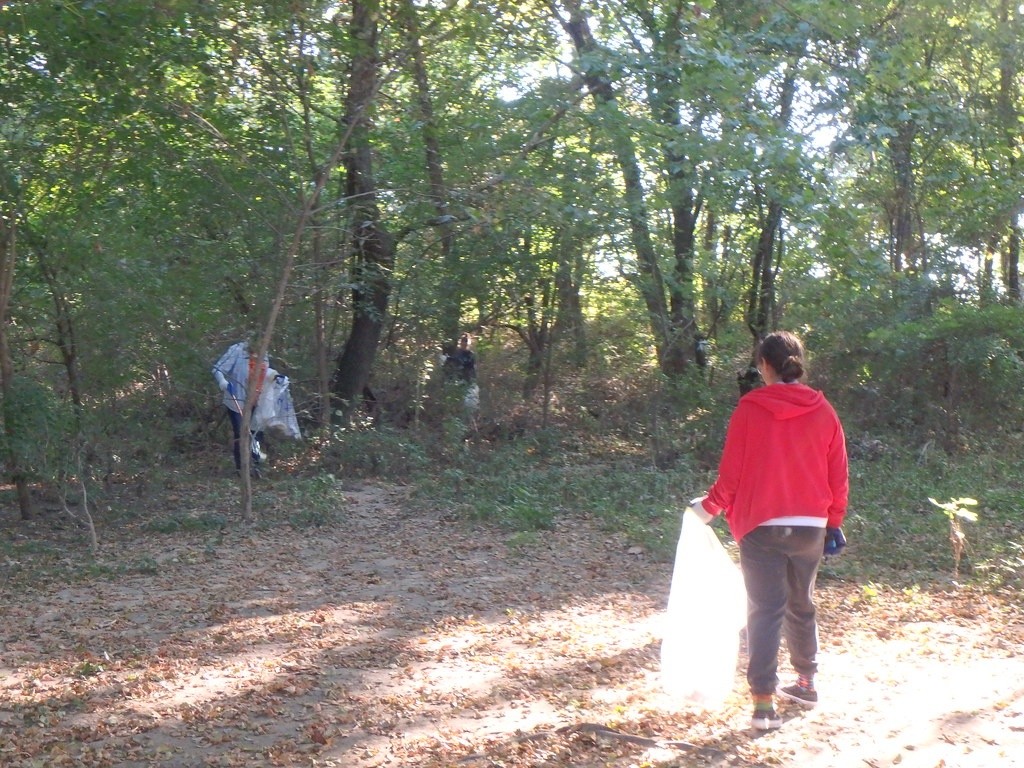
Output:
[227,383,234,395]
[275,375,284,384]
[823,526,844,556]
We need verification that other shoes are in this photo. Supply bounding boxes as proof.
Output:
[780,684,817,707]
[752,708,784,729]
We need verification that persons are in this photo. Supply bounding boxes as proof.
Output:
[211,329,270,477]
[689,330,852,730]
[444,336,478,383]
